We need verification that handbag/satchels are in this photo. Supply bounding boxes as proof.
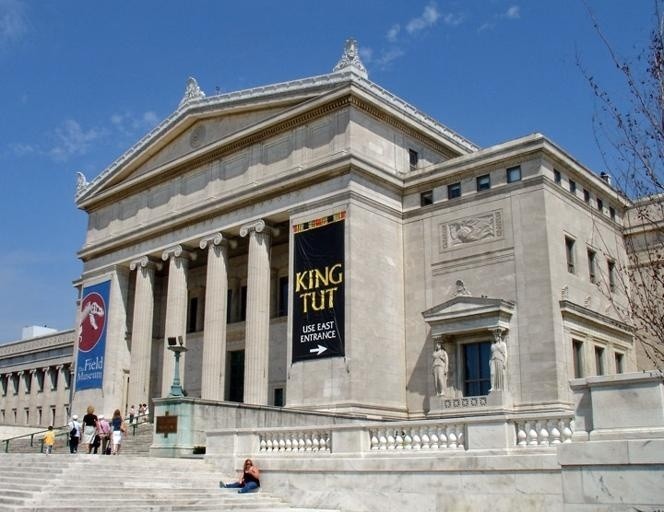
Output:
[70,428,77,436]
[121,422,127,433]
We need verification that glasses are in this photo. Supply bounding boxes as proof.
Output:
[247,463,252,465]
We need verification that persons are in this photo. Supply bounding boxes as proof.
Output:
[488,334,508,394]
[43,403,148,455]
[431,342,448,397]
[219,459,260,493]
